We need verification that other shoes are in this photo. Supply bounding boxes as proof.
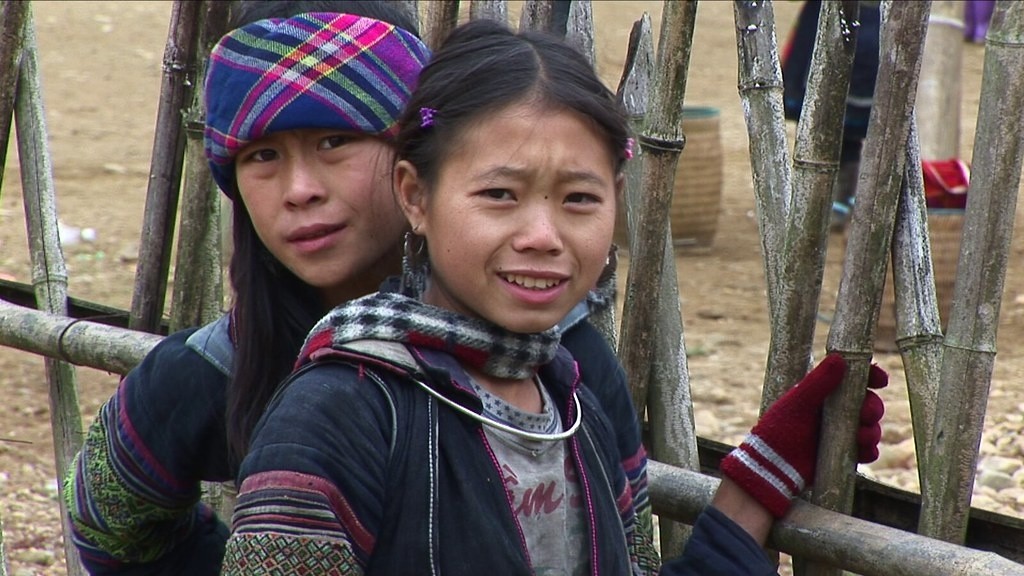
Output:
[831,193,857,228]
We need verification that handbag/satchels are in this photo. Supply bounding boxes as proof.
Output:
[921,157,972,209]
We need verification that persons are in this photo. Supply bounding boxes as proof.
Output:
[219,19,888,576]
[62,0,430,576]
[779,0,995,234]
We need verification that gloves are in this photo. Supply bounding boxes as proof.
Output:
[720,354,889,518]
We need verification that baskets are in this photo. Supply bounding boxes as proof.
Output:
[613,107,722,250]
